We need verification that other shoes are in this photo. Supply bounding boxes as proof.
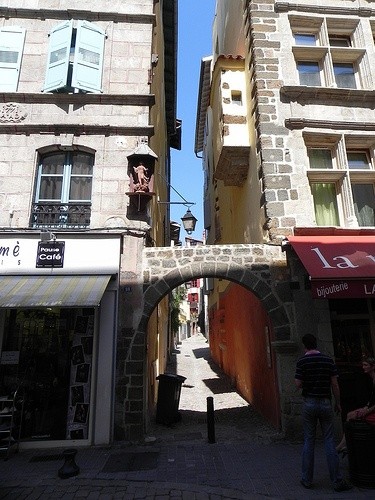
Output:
[300,480,313,489]
[334,482,354,492]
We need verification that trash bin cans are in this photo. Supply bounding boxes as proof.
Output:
[157,373,185,430]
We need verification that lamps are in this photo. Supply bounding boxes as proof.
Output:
[156,201,198,235]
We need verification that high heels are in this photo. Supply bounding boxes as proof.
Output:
[335,445,348,458]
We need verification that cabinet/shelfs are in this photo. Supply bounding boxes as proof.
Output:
[0,395,24,461]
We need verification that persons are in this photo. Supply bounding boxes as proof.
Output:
[336,355,375,460]
[292,334,346,489]
[132,162,150,185]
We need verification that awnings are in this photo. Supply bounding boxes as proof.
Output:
[0,273,112,307]
[283,234,375,300]
